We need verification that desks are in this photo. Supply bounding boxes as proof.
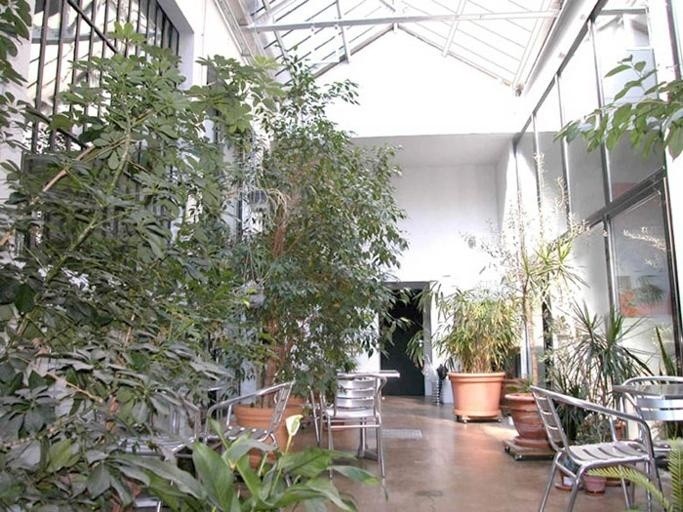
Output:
[295,369,399,445]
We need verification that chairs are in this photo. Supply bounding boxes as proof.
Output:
[525,384,667,511]
[618,373,681,510]
[318,374,388,479]
[118,374,295,508]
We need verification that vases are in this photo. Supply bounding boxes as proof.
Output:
[580,473,606,495]
[285,385,324,414]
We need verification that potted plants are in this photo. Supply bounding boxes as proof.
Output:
[463,154,592,460]
[429,288,522,423]
[231,56,360,467]
